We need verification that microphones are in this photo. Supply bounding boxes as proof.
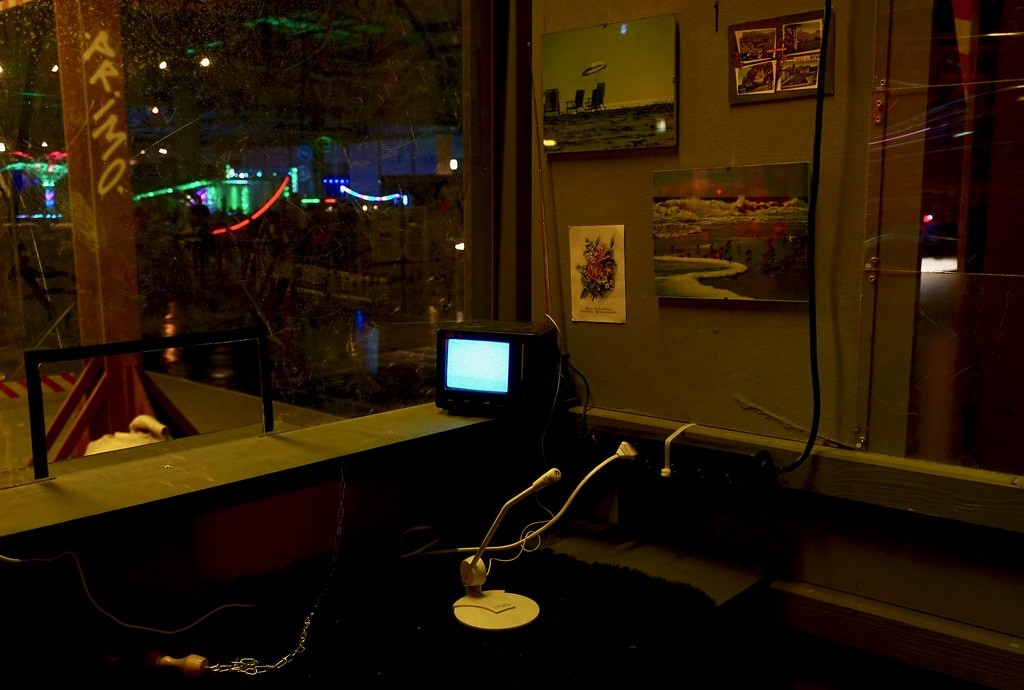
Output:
[453,467,562,630]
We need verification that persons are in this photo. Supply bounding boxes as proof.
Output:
[918,180,988,276]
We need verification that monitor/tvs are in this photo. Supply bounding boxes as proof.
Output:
[435,320,558,420]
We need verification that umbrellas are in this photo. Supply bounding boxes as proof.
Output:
[582,61,607,83]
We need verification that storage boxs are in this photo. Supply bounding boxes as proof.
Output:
[404,263,446,315]
[402,206,444,262]
[362,205,401,261]
[364,264,404,321]
